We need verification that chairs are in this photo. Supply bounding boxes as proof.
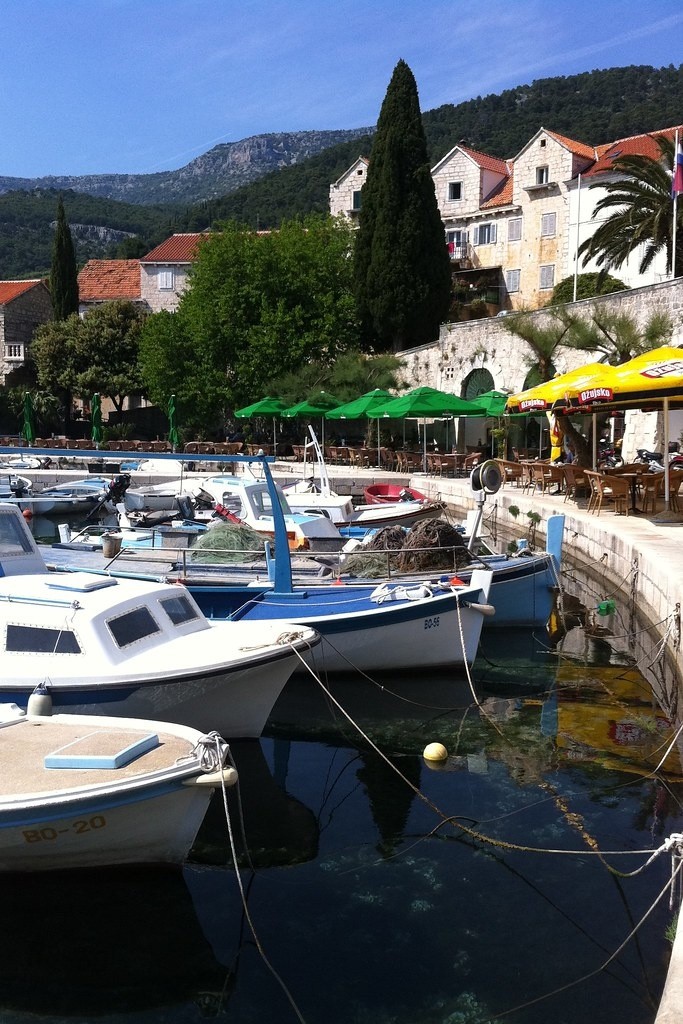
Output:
[292,444,683,517]
[0,437,29,447]
[108,440,167,453]
[184,441,279,457]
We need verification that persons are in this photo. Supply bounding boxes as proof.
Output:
[564,435,573,464]
[526,418,540,448]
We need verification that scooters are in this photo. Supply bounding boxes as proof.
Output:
[581,434,625,470]
[633,440,683,474]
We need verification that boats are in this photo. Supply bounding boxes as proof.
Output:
[0,424,567,746]
[262,663,563,777]
[0,860,234,1024]
[177,772,324,872]
[0,678,239,877]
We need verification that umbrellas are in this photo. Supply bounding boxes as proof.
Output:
[168,394,179,453]
[366,386,487,472]
[566,346,683,501]
[503,411,547,458]
[235,395,296,456]
[453,389,509,458]
[23,391,35,443]
[506,362,615,469]
[90,393,102,449]
[281,391,345,455]
[325,388,396,467]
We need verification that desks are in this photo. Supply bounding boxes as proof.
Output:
[615,472,654,514]
[548,463,573,496]
[444,454,467,477]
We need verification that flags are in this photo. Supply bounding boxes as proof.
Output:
[671,135,683,199]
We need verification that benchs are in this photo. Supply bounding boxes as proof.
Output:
[36,439,97,450]
[375,494,401,501]
